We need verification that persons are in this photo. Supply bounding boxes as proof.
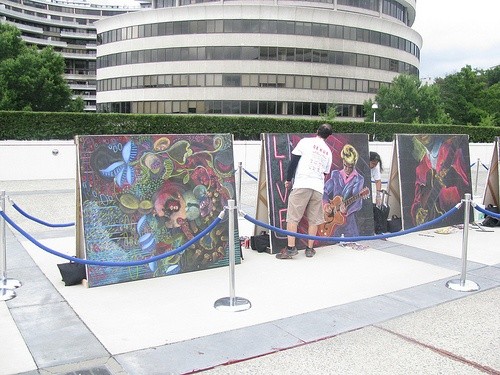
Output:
[370,152,388,240]
[276,124,332,259]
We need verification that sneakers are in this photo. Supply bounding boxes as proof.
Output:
[305,248,316,257]
[276,246,299,258]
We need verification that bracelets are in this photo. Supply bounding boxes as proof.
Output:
[285,181,290,184]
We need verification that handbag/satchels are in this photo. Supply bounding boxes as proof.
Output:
[388,215,402,233]
[58,256,86,286]
[250,230,269,252]
